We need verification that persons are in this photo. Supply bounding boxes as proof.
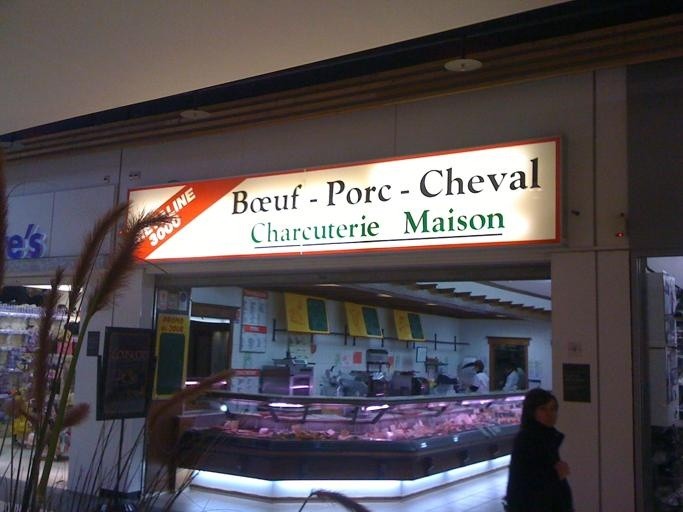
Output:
[428,360,525,396]
[503,388,574,512]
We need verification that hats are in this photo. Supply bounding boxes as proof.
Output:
[474,360,483,369]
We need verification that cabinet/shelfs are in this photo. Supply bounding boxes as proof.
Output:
[647,270,679,427]
